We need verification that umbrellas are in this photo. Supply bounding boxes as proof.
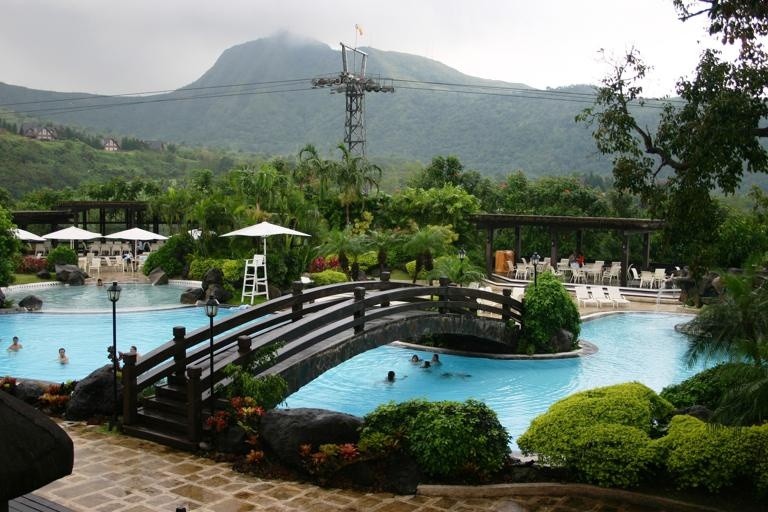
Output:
[184,225,215,240]
[218,218,313,303]
[42,223,103,250]
[8,226,47,242]
[98,226,170,271]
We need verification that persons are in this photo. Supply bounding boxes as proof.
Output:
[666,261,677,277]
[57,347,69,365]
[96,279,103,288]
[410,354,419,363]
[420,360,432,368]
[143,242,151,250]
[129,345,141,363]
[123,251,133,260]
[387,372,408,381]
[431,354,441,366]
[9,336,22,353]
[575,253,585,267]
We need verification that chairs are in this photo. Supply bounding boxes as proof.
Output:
[75,240,165,276]
[240,255,269,304]
[505,257,680,309]
[35,241,53,260]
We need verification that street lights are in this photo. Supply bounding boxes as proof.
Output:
[205,295,220,434]
[107,281,121,423]
[532,251,541,290]
